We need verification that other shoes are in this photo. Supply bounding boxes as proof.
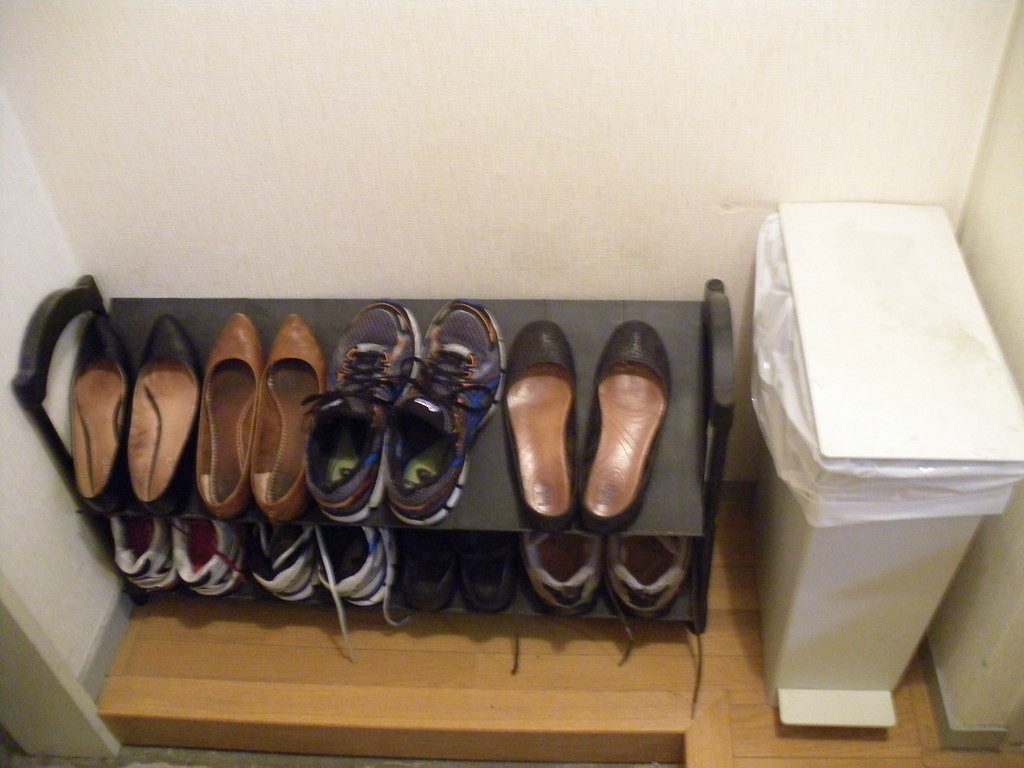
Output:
[109,516,704,720]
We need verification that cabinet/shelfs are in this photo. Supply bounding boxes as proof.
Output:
[12,271,734,637]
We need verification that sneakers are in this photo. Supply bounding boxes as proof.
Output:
[382,300,506,527]
[300,300,423,523]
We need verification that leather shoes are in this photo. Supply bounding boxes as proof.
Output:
[128,313,200,517]
[582,318,672,533]
[501,320,578,532]
[198,313,266,520]
[251,312,326,522]
[69,314,132,514]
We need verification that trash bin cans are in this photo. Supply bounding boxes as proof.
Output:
[750,201,1024,727]
